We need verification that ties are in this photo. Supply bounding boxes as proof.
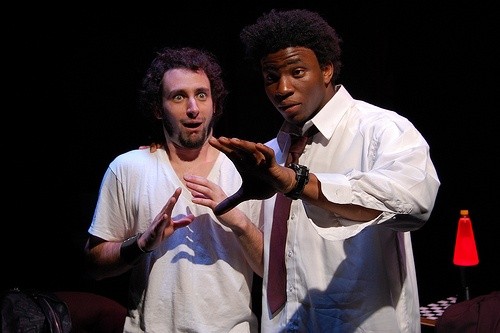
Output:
[267,124,320,314]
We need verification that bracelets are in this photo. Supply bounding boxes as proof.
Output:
[120,233,152,263]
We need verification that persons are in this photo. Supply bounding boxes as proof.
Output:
[84,47,265,333]
[208,7,441,333]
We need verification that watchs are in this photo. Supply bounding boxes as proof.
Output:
[284,161,310,200]
